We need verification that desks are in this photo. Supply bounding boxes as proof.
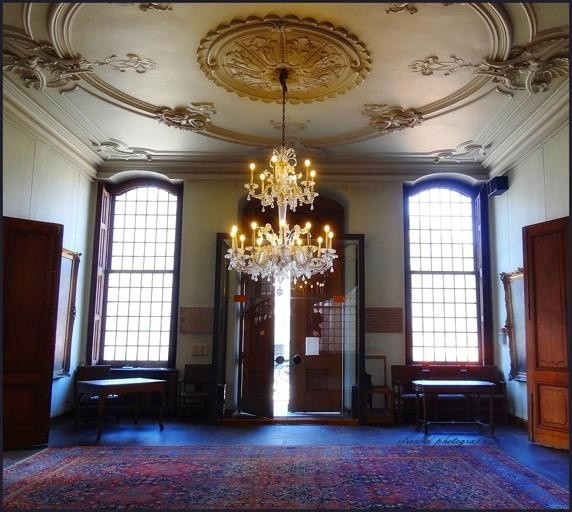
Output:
[76,376,167,443]
[411,378,496,437]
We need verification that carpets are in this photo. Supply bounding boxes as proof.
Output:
[1,444,569,510]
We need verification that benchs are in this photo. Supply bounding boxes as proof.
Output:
[391,363,508,424]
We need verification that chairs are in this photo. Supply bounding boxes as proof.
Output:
[72,365,120,425]
[178,362,213,420]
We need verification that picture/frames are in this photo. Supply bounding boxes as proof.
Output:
[51,246,82,379]
[498,266,527,384]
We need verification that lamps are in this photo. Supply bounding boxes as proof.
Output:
[225,65,339,287]
